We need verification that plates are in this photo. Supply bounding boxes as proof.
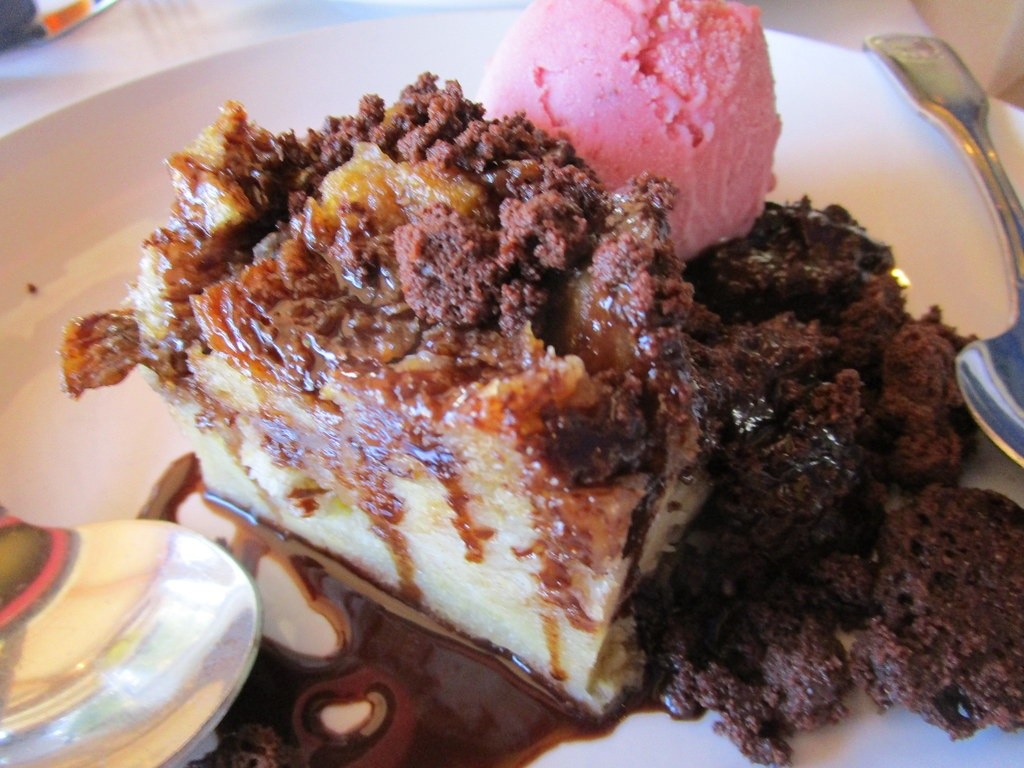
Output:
[0,12,1024,768]
[0,517,259,768]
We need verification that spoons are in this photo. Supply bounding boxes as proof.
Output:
[864,33,1024,468]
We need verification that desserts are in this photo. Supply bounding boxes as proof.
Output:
[54,0,1024,767]
